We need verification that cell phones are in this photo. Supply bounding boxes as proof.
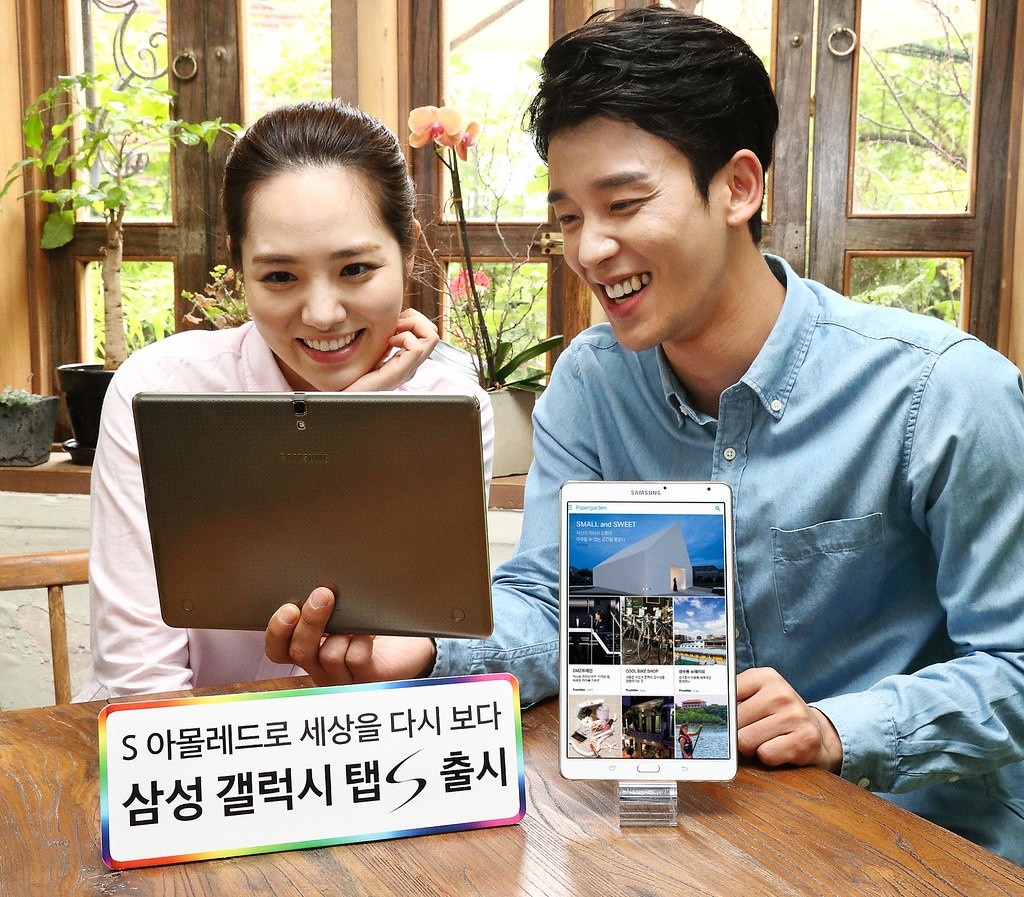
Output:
[560,481,739,782]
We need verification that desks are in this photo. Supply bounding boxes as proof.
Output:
[0,674,1024,897]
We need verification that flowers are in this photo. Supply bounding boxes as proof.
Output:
[406,106,563,395]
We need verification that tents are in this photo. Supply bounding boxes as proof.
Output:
[594,522,692,595]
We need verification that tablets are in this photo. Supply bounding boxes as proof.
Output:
[133,392,495,642]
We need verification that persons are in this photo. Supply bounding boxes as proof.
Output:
[673,578,678,591]
[265,7,1024,867]
[595,610,606,664]
[678,723,702,758]
[89,101,496,698]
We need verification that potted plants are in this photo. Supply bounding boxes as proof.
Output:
[489,394,538,479]
[0,70,242,465]
